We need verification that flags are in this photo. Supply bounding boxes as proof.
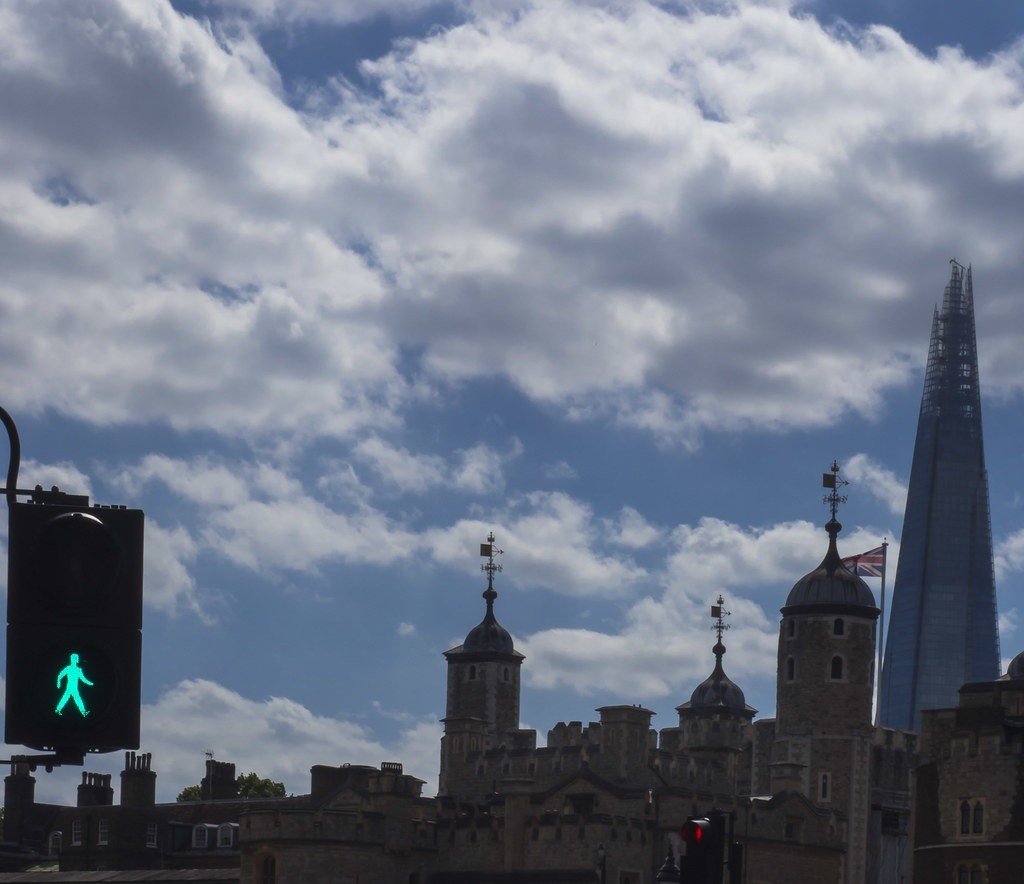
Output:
[840,545,884,578]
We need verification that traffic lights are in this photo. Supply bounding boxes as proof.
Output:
[5,501,142,753]
[677,813,727,884]
